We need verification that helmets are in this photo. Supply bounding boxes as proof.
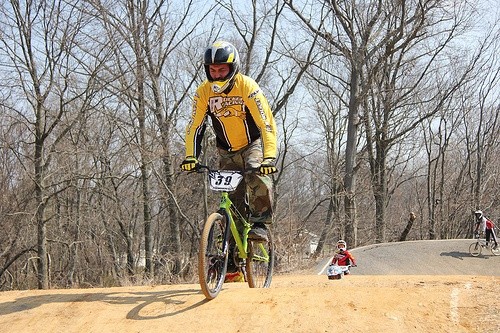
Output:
[475,210,484,220]
[216,233,222,254]
[202,41,240,95]
[336,240,347,252]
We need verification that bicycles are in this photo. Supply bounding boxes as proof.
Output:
[184,164,280,300]
[327,263,358,278]
[468,231,500,257]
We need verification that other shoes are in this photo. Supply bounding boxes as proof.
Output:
[248,221,270,242]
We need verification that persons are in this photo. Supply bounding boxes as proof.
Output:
[329,239,357,275]
[474,210,499,250]
[179,41,279,273]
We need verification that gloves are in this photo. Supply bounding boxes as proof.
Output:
[259,158,276,177]
[181,156,199,175]
